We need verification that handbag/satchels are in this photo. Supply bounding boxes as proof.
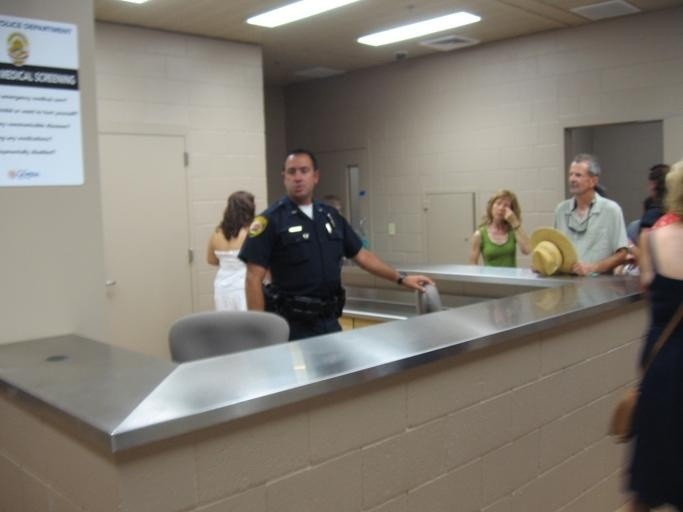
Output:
[613,391,641,441]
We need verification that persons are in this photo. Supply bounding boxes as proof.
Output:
[551,152,631,277]
[488,295,522,331]
[236,148,436,344]
[637,163,672,232]
[205,189,256,312]
[624,157,683,512]
[466,189,531,268]
[324,193,344,217]
[622,195,654,248]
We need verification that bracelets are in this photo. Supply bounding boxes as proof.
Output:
[396,269,408,286]
[512,223,521,230]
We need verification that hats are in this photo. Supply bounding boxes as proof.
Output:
[530,228,576,276]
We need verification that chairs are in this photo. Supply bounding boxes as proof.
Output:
[167,309,291,364]
[415,282,454,314]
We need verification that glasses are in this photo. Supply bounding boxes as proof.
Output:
[567,214,588,233]
